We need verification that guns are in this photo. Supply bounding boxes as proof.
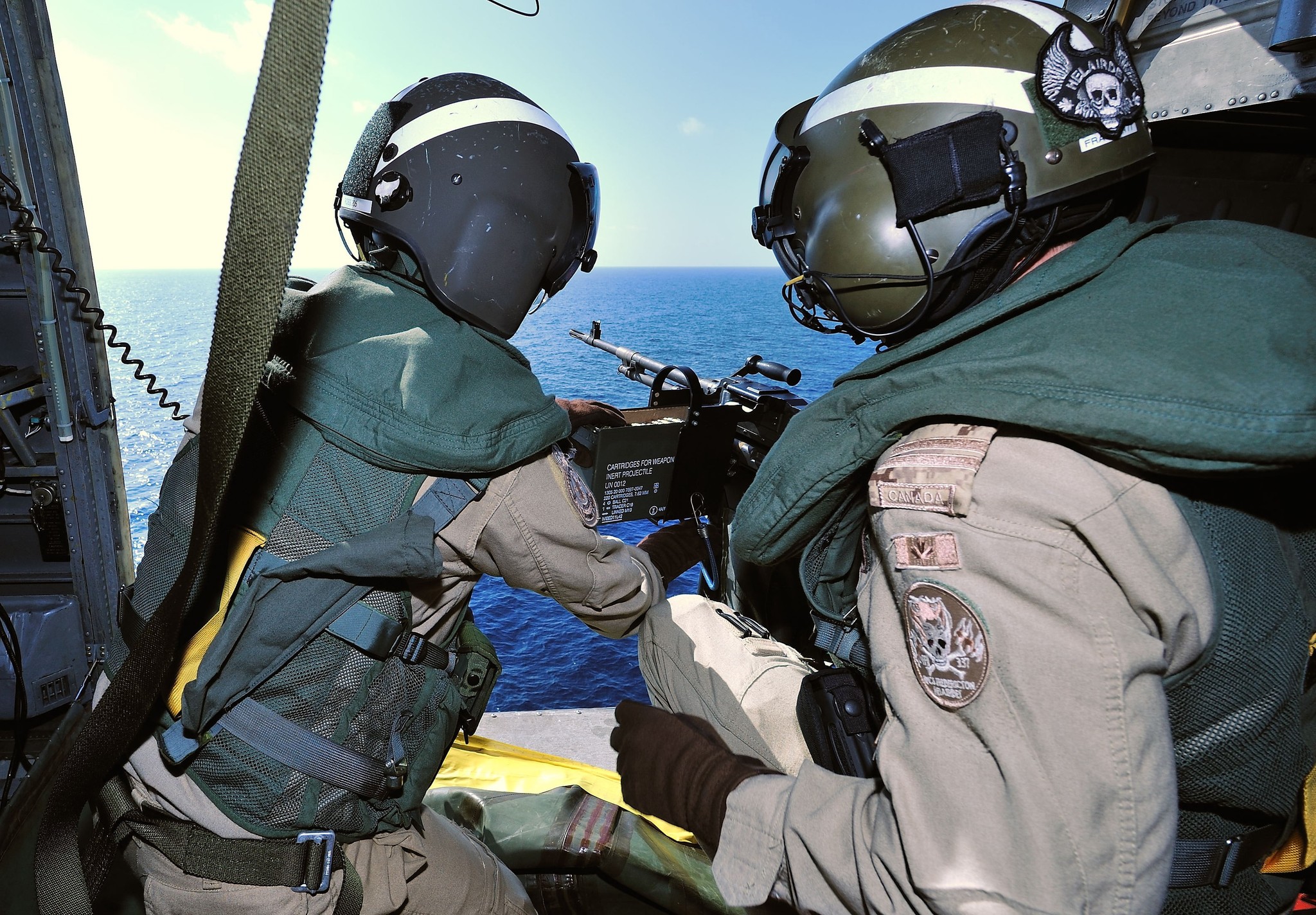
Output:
[570,321,810,581]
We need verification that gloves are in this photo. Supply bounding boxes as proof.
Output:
[609,697,788,863]
[554,397,626,431]
[635,517,723,592]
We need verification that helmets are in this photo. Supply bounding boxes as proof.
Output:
[751,0,1162,353]
[333,72,602,341]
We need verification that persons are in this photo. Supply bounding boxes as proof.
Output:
[605,2,1316,915]
[80,72,708,913]
